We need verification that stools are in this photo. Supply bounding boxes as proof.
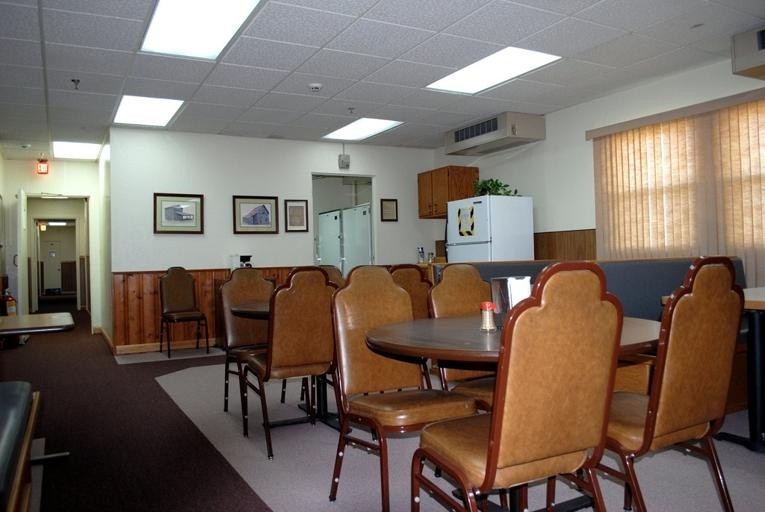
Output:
[1,378,45,511]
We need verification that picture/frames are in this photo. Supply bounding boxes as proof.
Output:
[380,198,398,222]
[232,194,280,234]
[153,192,205,234]
[284,199,309,232]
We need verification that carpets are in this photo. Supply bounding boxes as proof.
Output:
[114,345,227,365]
[152,359,763,512]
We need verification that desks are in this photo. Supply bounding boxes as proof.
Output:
[660,285,765,453]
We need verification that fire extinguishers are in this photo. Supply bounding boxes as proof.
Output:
[5,287,17,317]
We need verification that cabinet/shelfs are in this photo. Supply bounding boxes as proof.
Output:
[418,165,480,219]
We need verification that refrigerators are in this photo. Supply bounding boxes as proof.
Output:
[444,195,534,263]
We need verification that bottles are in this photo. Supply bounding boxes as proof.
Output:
[415,246,436,266]
[479,301,498,333]
